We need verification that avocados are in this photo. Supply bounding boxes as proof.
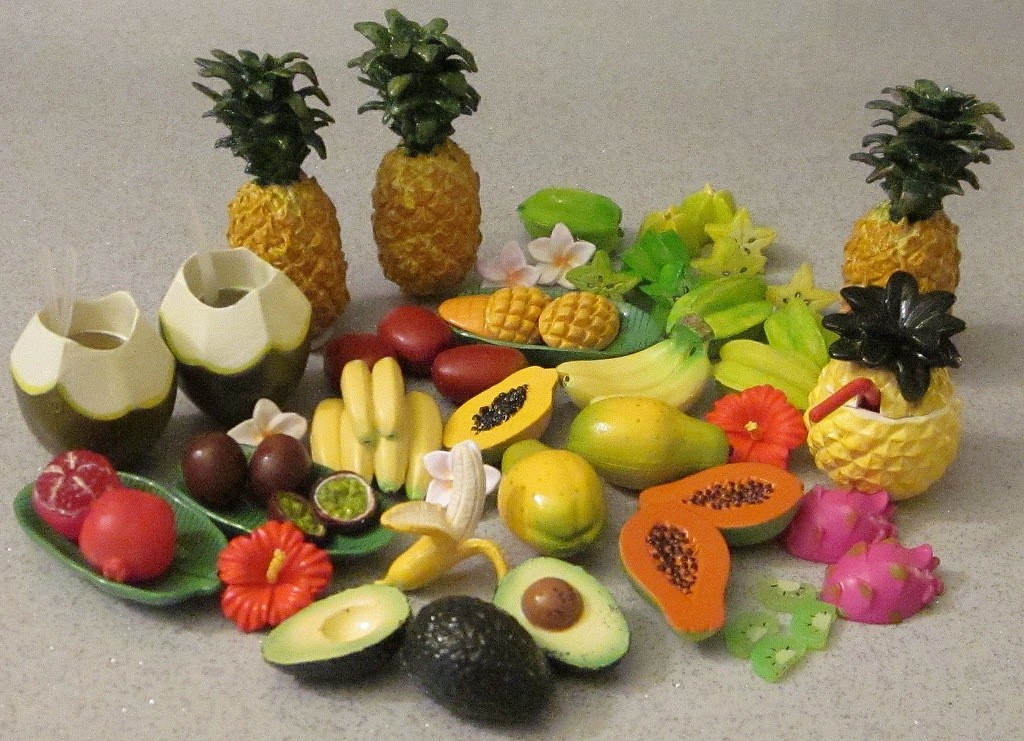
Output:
[262,583,414,681]
[401,595,551,723]
[491,556,630,671]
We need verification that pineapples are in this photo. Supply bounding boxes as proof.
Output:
[190,48,351,334]
[347,9,485,295]
[840,79,1016,316]
[800,269,966,505]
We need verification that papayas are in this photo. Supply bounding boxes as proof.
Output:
[442,365,558,456]
[498,439,607,557]
[567,398,731,489]
[634,462,804,548]
[618,504,732,642]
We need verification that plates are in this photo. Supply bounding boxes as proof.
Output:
[13,472,227,605]
[450,288,662,360]
[173,443,396,555]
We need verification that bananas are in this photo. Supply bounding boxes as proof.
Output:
[380,439,506,588]
[557,312,715,411]
[309,356,440,502]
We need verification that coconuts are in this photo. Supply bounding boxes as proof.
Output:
[7,288,178,470]
[157,247,315,421]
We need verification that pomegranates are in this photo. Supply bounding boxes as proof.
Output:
[31,450,177,583]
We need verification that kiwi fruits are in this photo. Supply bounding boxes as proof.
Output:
[722,578,838,682]
